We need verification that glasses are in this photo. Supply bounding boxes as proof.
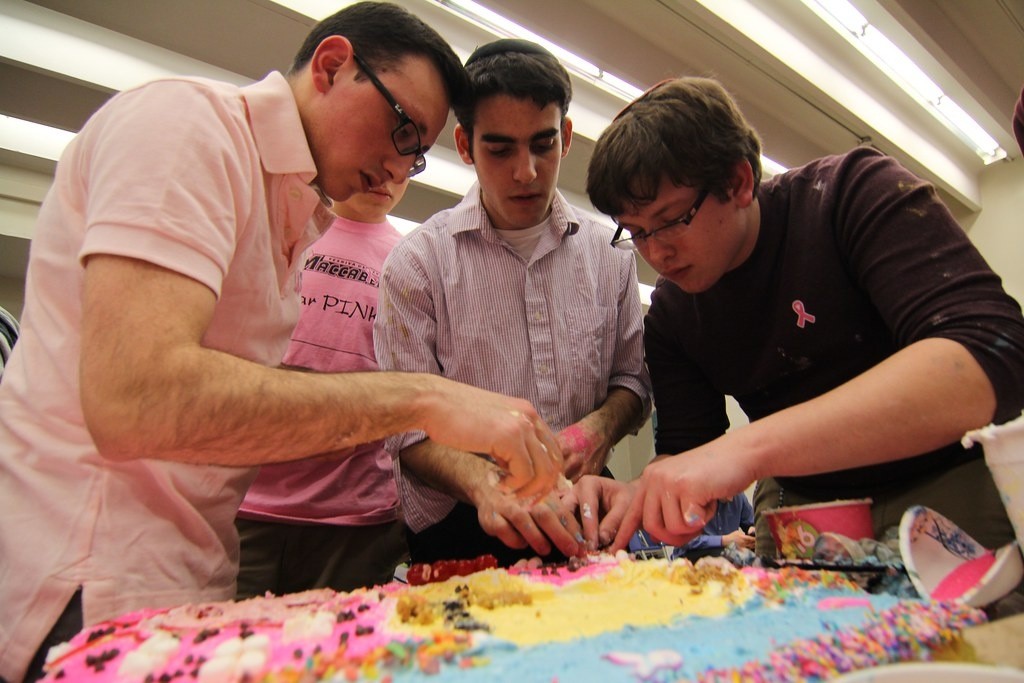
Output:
[352,51,427,179]
[608,185,711,251]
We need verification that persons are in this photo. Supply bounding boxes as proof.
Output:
[0,2,566,683]
[369,36,651,588]
[234,172,411,601]
[554,74,1024,619]
[672,491,758,569]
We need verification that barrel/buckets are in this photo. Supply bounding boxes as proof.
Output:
[757,497,875,567]
